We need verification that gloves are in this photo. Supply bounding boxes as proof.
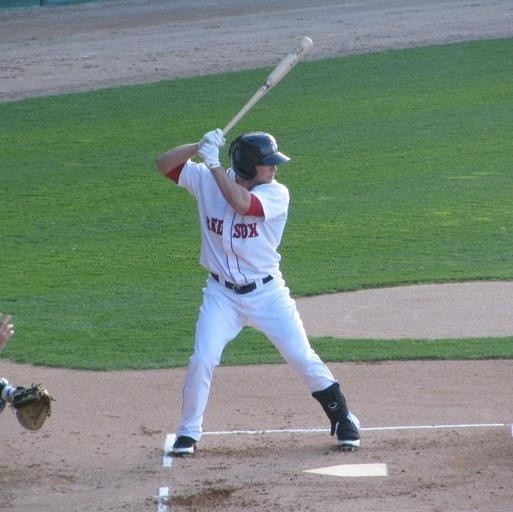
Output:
[196,128,226,171]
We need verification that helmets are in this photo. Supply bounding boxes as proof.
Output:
[227,132,291,179]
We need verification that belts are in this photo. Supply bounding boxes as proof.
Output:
[209,272,273,294]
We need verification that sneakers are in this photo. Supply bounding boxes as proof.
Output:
[171,436,197,455]
[335,412,361,452]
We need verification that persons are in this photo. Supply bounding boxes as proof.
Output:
[155,127,362,458]
[0,314,56,431]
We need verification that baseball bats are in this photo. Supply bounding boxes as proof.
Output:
[222,36,313,135]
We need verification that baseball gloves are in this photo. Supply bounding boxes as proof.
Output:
[12,382,51,431]
